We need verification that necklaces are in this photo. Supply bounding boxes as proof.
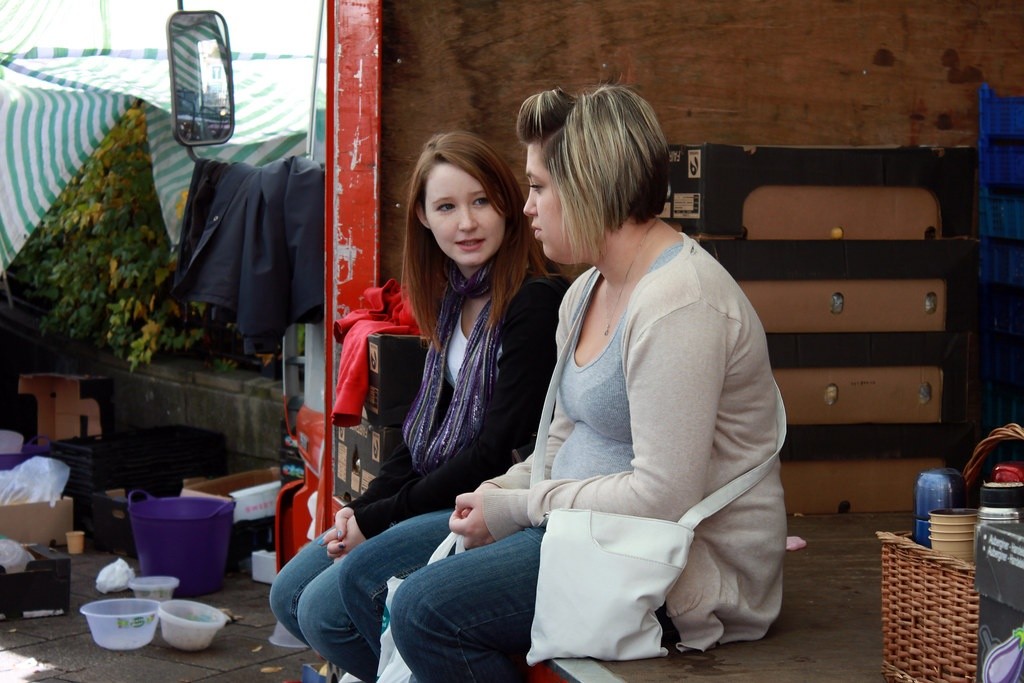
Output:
[603,219,658,333]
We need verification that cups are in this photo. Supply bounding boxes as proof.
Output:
[66,531,84,554]
[927,508,977,563]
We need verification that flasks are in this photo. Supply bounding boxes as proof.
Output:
[973,481,1024,565]
[913,469,965,547]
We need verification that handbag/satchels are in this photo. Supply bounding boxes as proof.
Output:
[527,509,695,667]
[376,532,465,683]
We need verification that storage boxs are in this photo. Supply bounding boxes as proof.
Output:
[653,140,979,517]
[0,392,283,621]
[364,327,434,425]
[332,416,402,505]
[977,522,1024,683]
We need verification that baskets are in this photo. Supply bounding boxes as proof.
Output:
[875,424,1024,683]
[50,424,230,540]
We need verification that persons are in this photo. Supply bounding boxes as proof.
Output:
[338,84,786,683]
[270,133,572,683]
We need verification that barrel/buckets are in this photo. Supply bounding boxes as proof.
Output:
[0,436,50,470]
[128,490,236,596]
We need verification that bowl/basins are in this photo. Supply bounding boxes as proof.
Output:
[128,577,180,603]
[158,599,227,651]
[80,597,160,650]
